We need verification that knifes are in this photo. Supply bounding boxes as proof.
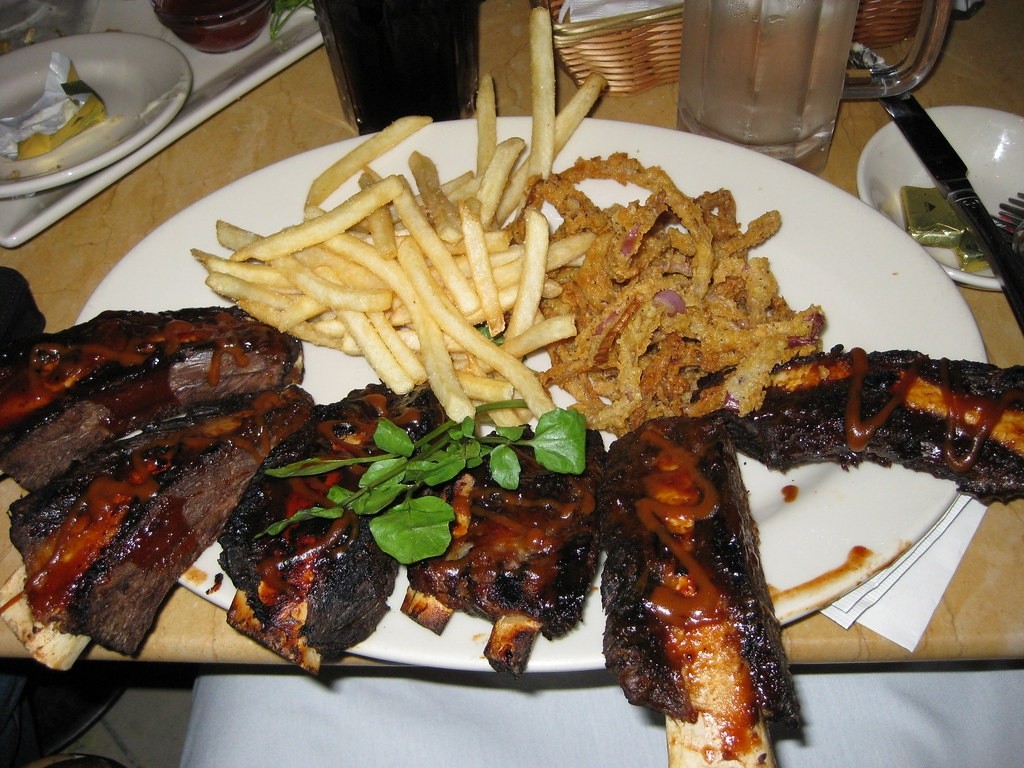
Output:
[848,42,1024,335]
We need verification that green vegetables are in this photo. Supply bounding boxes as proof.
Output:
[259,399,587,562]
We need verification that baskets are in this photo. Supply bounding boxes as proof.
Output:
[527,0,924,98]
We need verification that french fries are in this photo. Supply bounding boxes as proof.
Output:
[191,4,610,428]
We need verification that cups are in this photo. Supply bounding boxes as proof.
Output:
[312,0,482,135]
[677,0,955,174]
[150,0,273,54]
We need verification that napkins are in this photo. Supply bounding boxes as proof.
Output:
[817,491,986,654]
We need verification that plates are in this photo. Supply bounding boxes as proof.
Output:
[0,33,190,199]
[73,117,990,670]
[0,0,328,247]
[857,106,1024,290]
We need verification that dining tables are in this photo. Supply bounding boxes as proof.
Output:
[1,1,1024,669]
